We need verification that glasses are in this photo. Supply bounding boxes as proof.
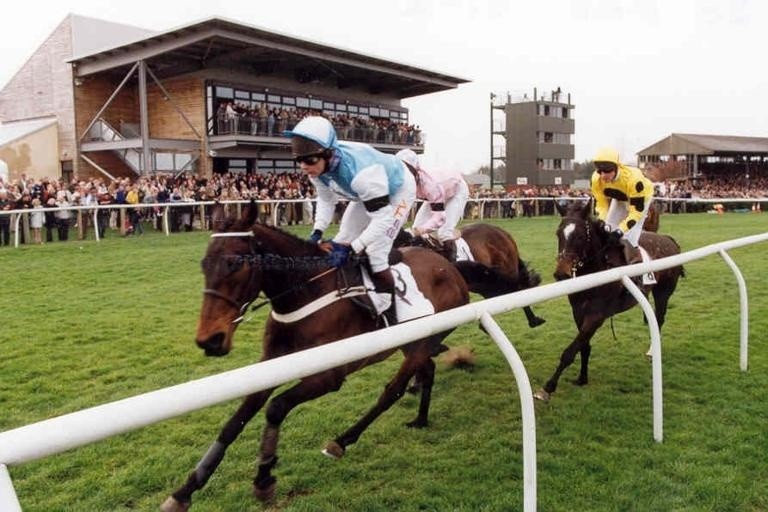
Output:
[297,148,330,166]
[597,167,614,174]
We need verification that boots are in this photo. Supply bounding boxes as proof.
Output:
[370,266,399,330]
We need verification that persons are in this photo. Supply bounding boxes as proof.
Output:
[282,114,417,328]
[216,100,421,146]
[1,171,317,246]
[588,149,654,263]
[463,186,592,219]
[392,147,471,263]
[656,172,768,215]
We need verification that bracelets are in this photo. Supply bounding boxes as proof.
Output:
[350,240,363,254]
[311,228,322,237]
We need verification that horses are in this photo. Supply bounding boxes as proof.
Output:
[383,222,541,394]
[532,197,685,405]
[643,203,661,233]
[161,196,543,512]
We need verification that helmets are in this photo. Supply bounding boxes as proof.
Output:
[592,148,621,166]
[282,116,337,156]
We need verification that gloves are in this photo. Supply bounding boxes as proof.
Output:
[331,245,352,268]
